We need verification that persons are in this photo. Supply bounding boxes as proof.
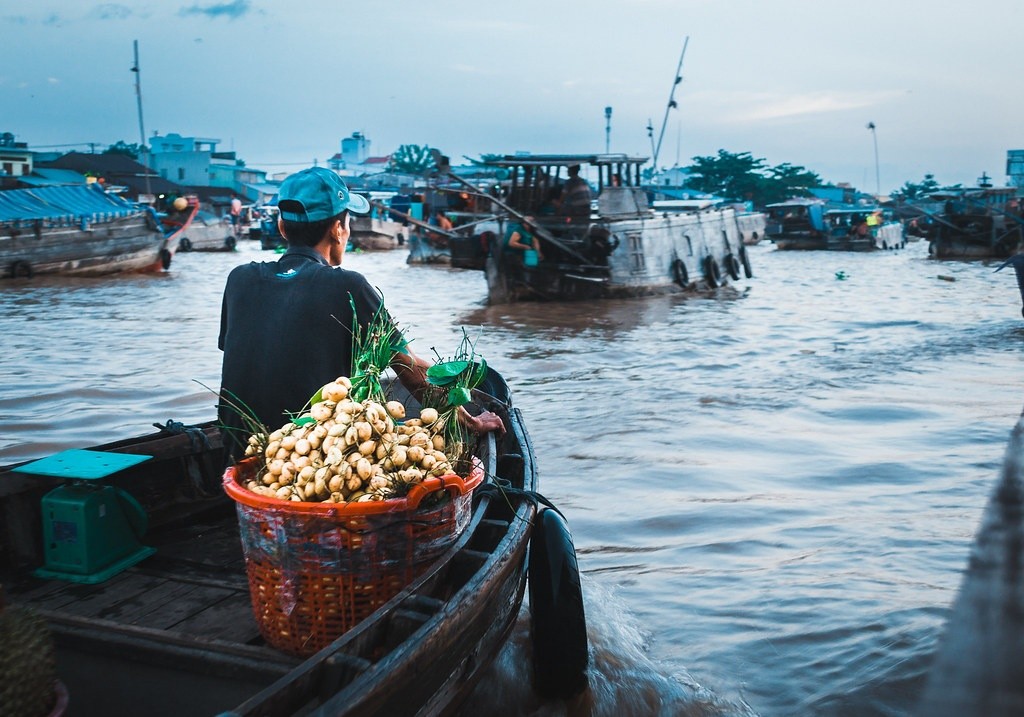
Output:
[551,164,592,219]
[426,209,458,248]
[225,190,243,237]
[501,216,544,294]
[214,168,507,469]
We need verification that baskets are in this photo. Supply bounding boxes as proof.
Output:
[224,447,485,658]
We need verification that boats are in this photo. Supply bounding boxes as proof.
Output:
[258,191,411,252]
[446,211,500,270]
[486,151,751,297]
[0,184,199,279]
[764,199,877,251]
[156,207,238,251]
[0,361,537,717]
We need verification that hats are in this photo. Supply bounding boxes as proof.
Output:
[523,216,541,228]
[277,166,371,224]
